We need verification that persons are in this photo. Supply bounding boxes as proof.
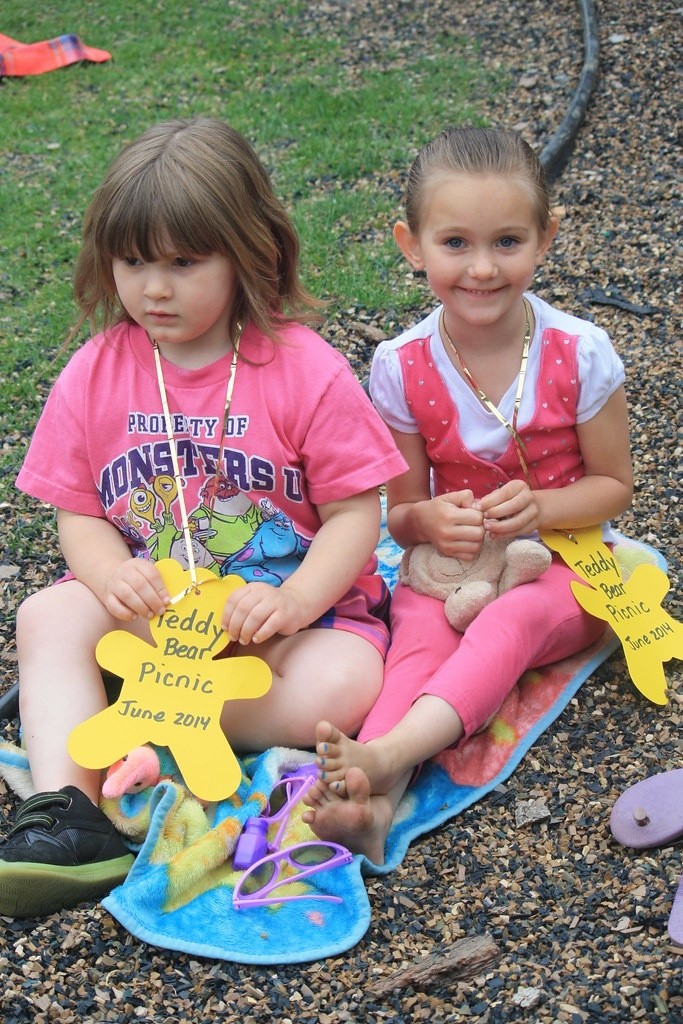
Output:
[0,118,410,918]
[301,126,634,865]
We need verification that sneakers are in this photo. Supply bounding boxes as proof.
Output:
[0,784,138,918]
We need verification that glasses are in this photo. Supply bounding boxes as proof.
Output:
[257,774,318,854]
[231,840,354,906]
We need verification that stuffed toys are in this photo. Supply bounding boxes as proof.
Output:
[102,742,181,799]
[398,499,552,632]
